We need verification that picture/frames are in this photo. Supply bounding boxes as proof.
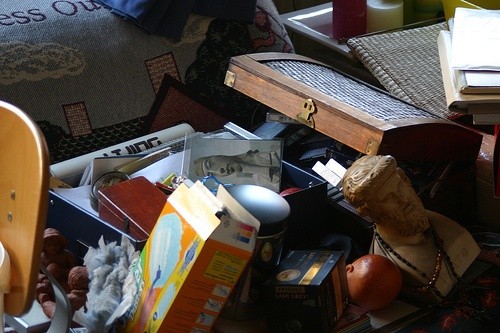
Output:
[178,132,283,202]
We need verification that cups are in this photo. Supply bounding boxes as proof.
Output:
[367,0,403,33]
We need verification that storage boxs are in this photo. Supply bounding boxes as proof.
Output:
[223,51,482,242]
[116,180,261,333]
[261,251,351,333]
[98,176,168,239]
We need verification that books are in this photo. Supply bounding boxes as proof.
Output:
[77,131,205,187]
[438,7,500,125]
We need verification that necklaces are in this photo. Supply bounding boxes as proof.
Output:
[369,219,477,317]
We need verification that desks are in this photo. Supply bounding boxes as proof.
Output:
[277,0,500,175]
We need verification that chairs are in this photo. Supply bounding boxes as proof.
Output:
[1,102,72,333]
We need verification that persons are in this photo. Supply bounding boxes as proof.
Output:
[340,154,481,311]
[193,147,282,192]
[36,228,92,331]
